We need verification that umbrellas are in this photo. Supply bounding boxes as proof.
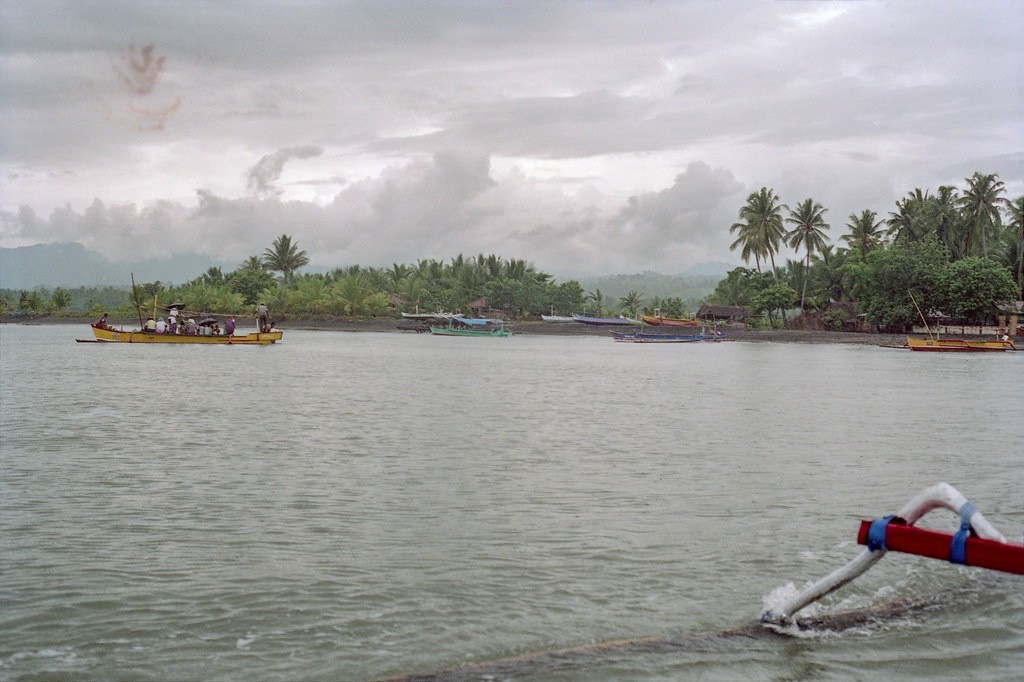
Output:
[166,303,186,311]
[198,316,218,326]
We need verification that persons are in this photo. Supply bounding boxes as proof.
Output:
[252,302,275,332]
[144,306,236,336]
[99,313,109,329]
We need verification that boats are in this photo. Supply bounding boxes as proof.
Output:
[540,314,575,323]
[400,305,516,337]
[608,311,734,344]
[639,312,701,327]
[571,311,637,326]
[74,272,285,344]
[878,290,1024,352]
